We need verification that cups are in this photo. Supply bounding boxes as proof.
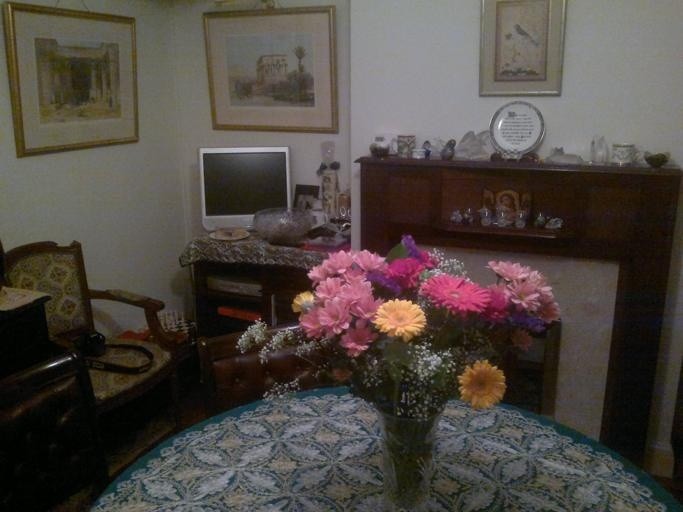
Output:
[449,204,559,232]
[391,134,415,158]
[410,148,426,159]
[612,142,636,167]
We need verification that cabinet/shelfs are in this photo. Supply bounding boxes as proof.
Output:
[176,219,354,350]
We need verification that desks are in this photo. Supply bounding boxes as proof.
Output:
[83,378,683,512]
[350,149,682,476]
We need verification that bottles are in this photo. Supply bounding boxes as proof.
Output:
[371,137,389,160]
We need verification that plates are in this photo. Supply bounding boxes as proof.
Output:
[488,101,545,152]
[209,229,250,241]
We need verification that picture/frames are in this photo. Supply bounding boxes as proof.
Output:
[0,1,141,159]
[199,4,341,136]
[476,0,569,100]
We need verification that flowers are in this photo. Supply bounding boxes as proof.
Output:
[222,229,566,510]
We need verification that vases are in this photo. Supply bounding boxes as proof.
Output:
[361,398,448,512]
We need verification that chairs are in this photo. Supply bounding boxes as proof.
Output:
[192,311,364,422]
[0,349,116,512]
[1,238,189,477]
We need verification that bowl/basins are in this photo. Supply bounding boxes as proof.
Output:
[254,207,313,244]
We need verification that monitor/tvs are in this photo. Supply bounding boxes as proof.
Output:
[198,146,292,231]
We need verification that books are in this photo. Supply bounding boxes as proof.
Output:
[218,306,262,322]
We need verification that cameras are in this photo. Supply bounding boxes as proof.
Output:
[57,328,107,357]
